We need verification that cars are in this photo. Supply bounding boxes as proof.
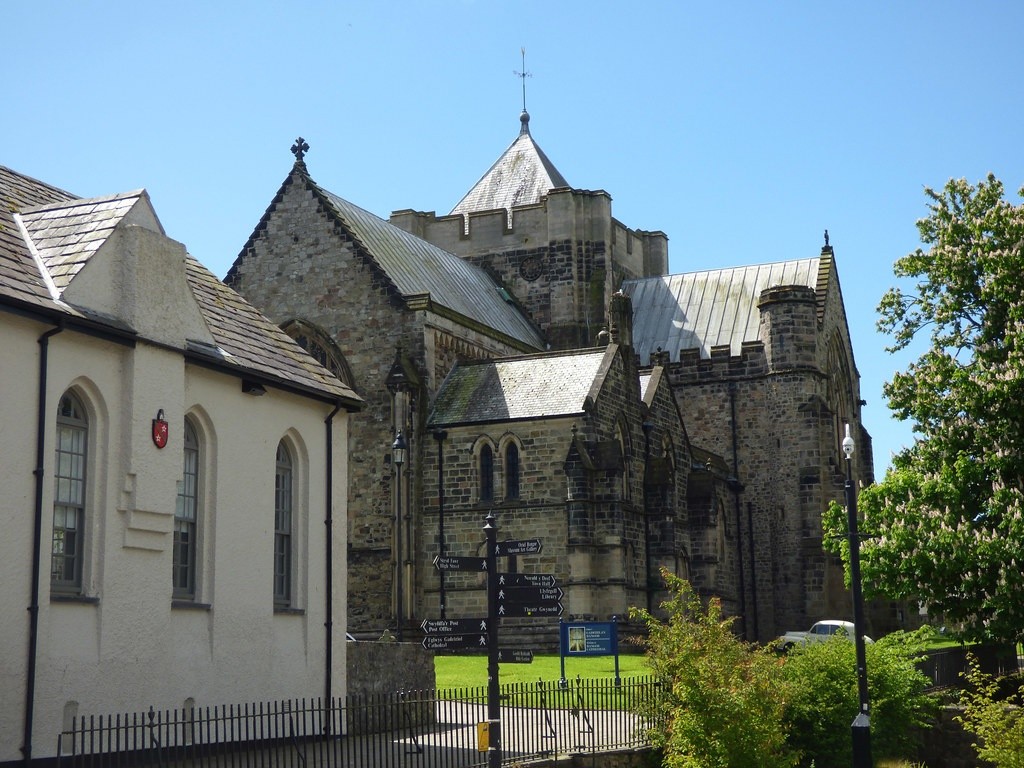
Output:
[777,620,876,655]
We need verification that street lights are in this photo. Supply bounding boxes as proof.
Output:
[388,429,408,641]
[842,422,873,767]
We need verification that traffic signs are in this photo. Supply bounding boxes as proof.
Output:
[497,648,534,664]
[419,617,489,637]
[432,555,487,572]
[498,587,564,605]
[495,538,543,557]
[498,602,564,618]
[421,633,489,651]
[498,574,557,588]
